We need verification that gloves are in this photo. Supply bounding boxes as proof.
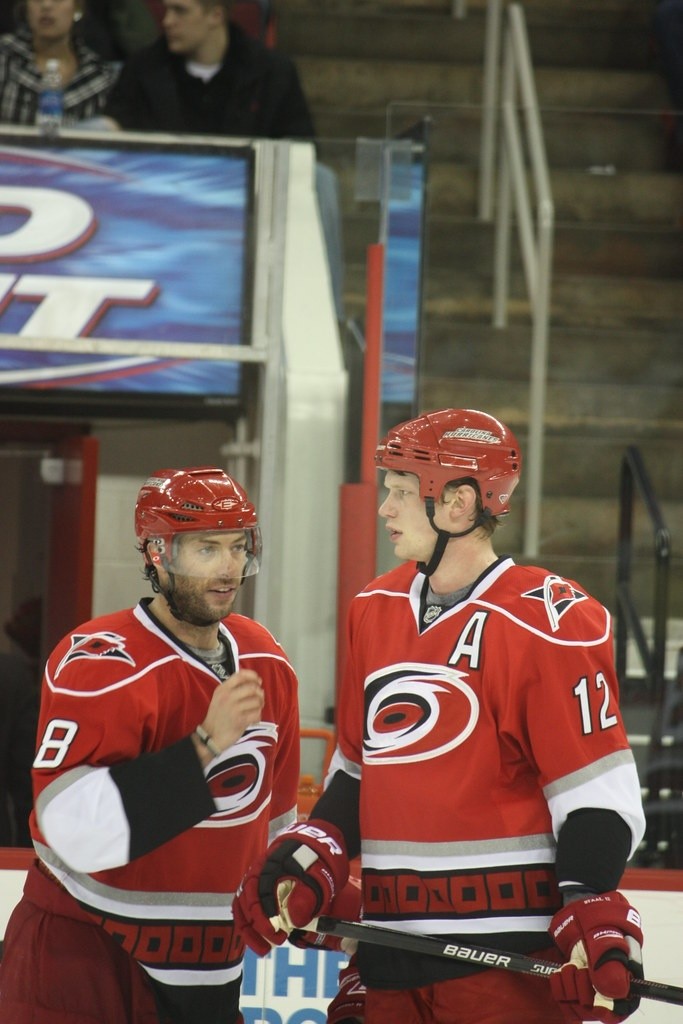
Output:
[547,890,644,1024]
[232,819,350,957]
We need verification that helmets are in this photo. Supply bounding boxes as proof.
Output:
[133,467,263,567]
[375,407,521,518]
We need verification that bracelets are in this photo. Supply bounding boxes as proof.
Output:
[194,725,223,761]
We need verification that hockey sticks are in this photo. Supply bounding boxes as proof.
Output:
[298,916,683,1007]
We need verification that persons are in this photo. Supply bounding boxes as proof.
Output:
[0,463,302,1024]
[229,406,652,1024]
[89,0,321,149]
[0,2,126,139]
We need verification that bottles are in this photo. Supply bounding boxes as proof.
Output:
[34,58,63,127]
